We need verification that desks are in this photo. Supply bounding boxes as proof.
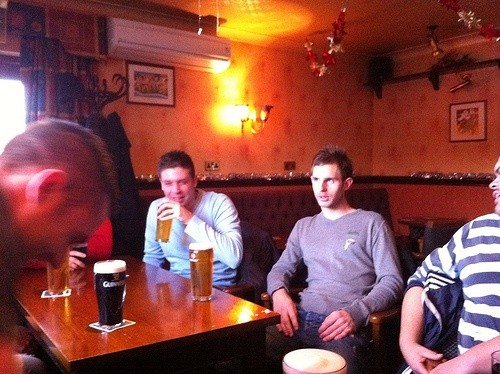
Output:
[398,217,468,243]
[10,255,282,374]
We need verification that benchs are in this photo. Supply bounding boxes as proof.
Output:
[138,188,393,237]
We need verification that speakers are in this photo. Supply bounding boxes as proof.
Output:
[365,56,393,84]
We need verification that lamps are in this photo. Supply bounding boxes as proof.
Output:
[237,103,273,134]
[450,80,470,92]
[430,37,443,56]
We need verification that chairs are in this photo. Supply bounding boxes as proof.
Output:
[226,220,500,374]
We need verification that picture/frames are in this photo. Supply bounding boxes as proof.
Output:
[125,60,176,107]
[449,100,487,143]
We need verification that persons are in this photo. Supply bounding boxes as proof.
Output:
[142,149,243,296]
[0,115,114,373]
[254,148,404,374]
[10,218,112,274]
[398,156,500,374]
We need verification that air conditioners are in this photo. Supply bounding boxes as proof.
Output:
[106,17,232,73]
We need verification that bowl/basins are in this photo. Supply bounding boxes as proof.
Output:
[282,348,347,373]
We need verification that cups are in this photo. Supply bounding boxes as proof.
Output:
[189,243,215,302]
[72,243,88,270]
[46,253,70,296]
[155,201,173,243]
[93,260,127,329]
